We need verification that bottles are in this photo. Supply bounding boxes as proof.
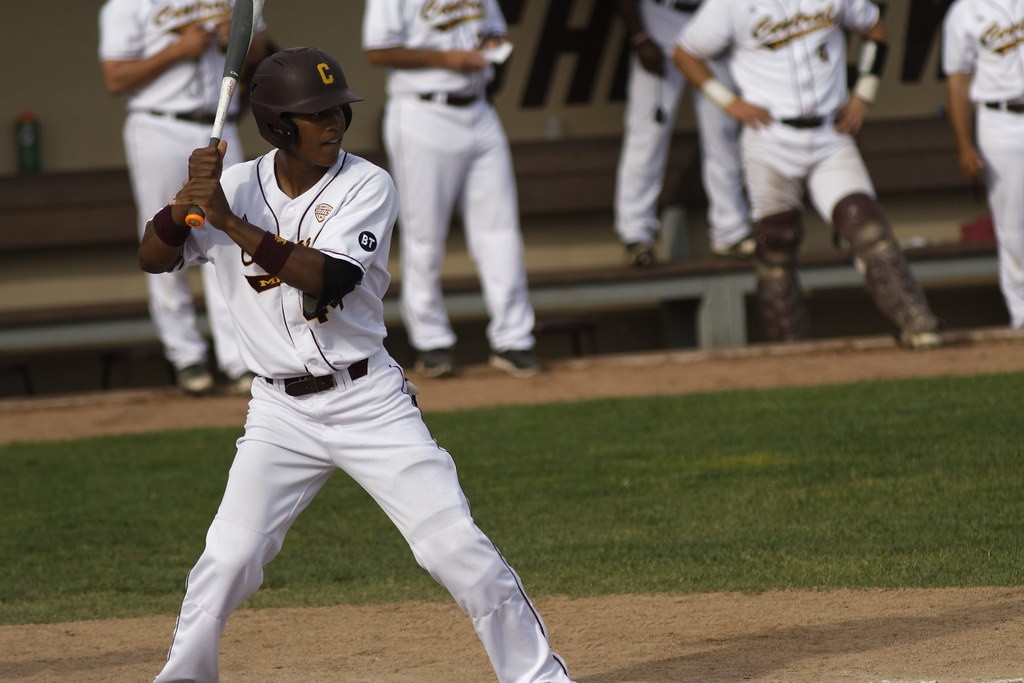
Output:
[15,112,41,176]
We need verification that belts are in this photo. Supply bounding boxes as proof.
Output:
[259,357,369,397]
[986,101,1024,115]
[151,108,237,125]
[784,115,840,129]
[421,90,476,107]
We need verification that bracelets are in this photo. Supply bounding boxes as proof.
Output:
[150,204,192,247]
[629,28,650,44]
[252,230,295,278]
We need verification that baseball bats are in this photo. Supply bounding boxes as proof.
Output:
[183,0,266,231]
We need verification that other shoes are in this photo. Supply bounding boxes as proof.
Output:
[413,347,453,378]
[233,370,258,396]
[178,366,214,395]
[624,240,655,269]
[489,348,543,379]
[718,233,756,258]
[899,325,943,349]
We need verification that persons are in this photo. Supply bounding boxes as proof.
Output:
[98,0,268,395]
[612,0,760,264]
[938,0,1024,328]
[134,48,576,683]
[362,0,551,378]
[673,0,941,337]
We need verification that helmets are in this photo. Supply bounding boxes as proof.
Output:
[249,46,365,150]
